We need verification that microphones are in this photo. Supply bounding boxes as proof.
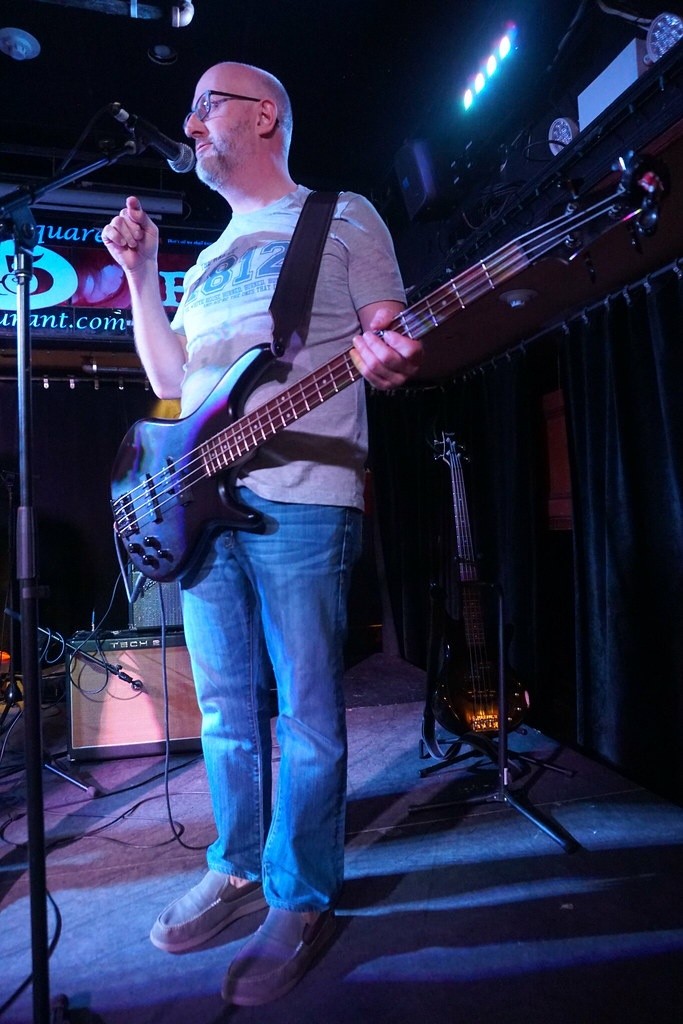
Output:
[110,103,195,173]
[45,0,195,27]
[119,672,143,690]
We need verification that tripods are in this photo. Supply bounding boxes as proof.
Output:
[0,470,100,798]
[407,555,579,856]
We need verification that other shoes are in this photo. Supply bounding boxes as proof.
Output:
[220,908,341,1005]
[150,869,267,951]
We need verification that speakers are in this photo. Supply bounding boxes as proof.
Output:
[62,624,203,762]
[127,561,183,629]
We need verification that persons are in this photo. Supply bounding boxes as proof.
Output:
[101,61,423,1007]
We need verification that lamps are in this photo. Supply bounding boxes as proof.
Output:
[647,13,683,63]
[548,118,579,156]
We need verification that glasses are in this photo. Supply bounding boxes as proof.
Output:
[184,90,279,129]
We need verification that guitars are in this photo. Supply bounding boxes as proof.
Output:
[104,149,665,585]
[421,422,541,743]
